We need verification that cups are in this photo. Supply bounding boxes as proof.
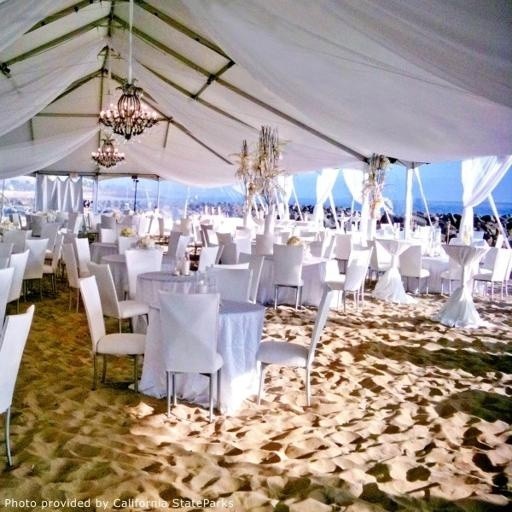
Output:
[393,223,400,239]
[171,260,191,276]
[456,225,483,246]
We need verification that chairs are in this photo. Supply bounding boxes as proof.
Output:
[0,208,512,467]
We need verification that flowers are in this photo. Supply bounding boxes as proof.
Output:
[228,140,257,210]
[361,153,389,207]
[249,126,294,210]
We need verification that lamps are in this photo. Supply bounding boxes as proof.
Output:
[98,0,172,141]
[91,46,126,168]
